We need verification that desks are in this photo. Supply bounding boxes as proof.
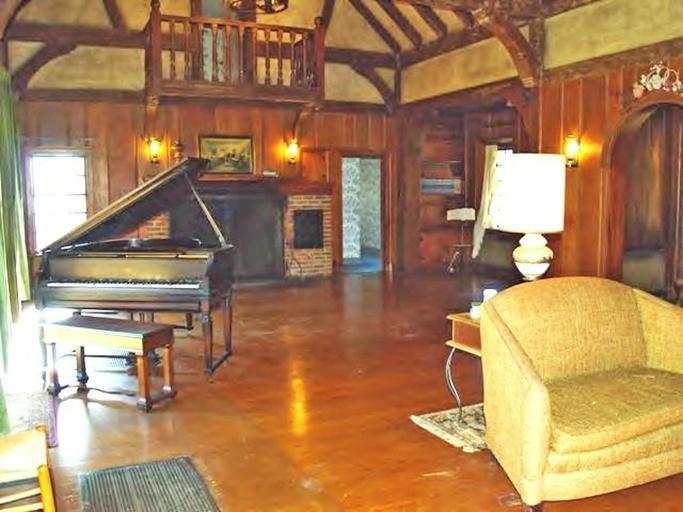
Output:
[169,180,284,285]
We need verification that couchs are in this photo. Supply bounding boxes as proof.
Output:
[480,275,683,511]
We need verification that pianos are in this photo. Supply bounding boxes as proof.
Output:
[33,157,234,377]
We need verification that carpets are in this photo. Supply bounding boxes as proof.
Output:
[77,457,221,512]
[410,401,489,453]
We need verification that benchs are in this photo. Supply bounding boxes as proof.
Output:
[41,314,177,412]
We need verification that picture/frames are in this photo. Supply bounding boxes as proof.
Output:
[198,134,255,175]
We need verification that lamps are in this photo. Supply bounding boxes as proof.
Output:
[148,139,161,164]
[484,153,565,280]
[286,140,300,167]
[447,207,476,244]
[563,135,580,169]
